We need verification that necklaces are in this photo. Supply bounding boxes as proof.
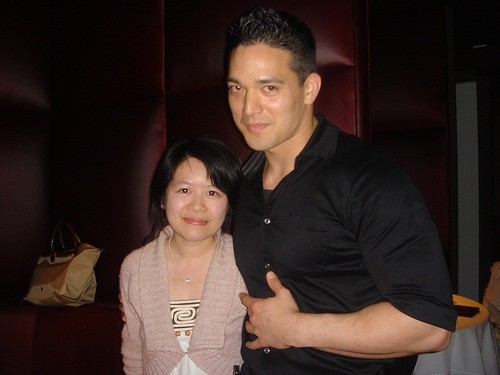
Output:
[169,234,219,284]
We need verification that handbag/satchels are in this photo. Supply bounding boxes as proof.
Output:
[23,222,103,306]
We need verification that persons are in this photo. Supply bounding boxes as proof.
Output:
[117,5,459,375]
[120,135,251,375]
